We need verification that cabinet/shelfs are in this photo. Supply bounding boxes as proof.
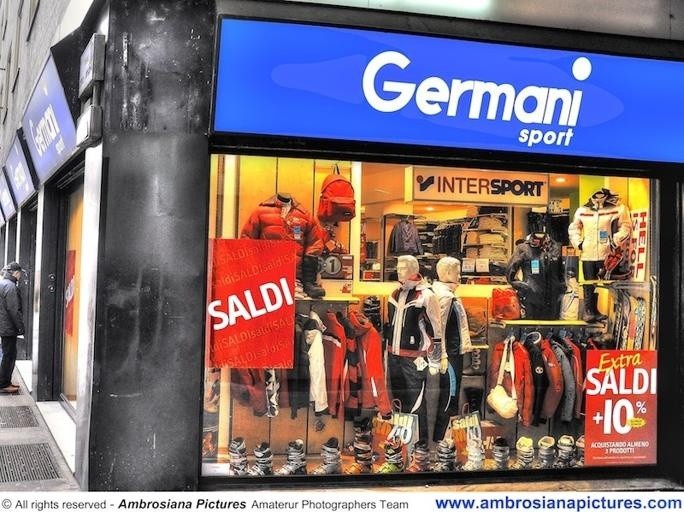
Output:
[386,213,512,279]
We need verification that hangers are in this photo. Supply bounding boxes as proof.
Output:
[295,301,363,325]
[497,325,603,348]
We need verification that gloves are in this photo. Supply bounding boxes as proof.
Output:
[15,328,25,335]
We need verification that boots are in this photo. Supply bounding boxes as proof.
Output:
[582,292,609,322]
[302,252,326,298]
[224,433,584,477]
[462,347,486,375]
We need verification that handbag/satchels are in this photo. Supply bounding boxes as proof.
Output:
[444,400,483,466]
[369,397,420,461]
[486,333,521,420]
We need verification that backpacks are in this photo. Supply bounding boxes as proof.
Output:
[316,162,357,222]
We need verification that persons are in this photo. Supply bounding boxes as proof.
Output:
[382,254,444,449]
[504,230,567,320]
[431,254,477,441]
[239,189,326,281]
[0,261,28,395]
[568,187,634,280]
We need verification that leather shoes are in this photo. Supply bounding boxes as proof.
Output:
[0,383,21,393]
